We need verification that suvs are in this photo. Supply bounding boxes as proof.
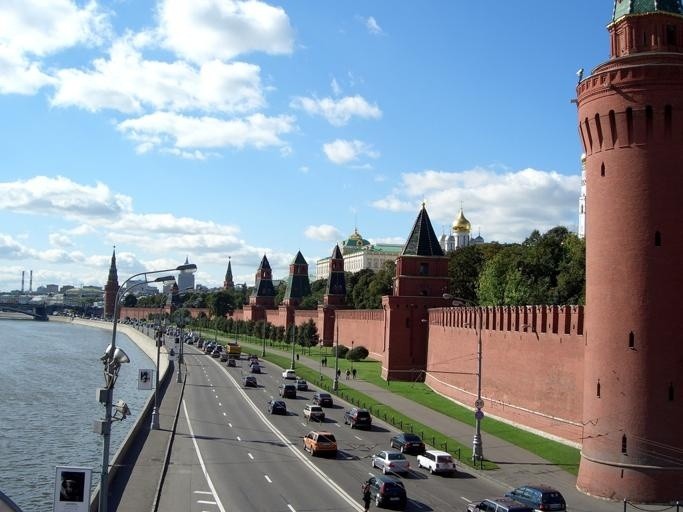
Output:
[342,407,372,429]
[277,382,296,399]
[357,475,408,507]
[301,430,338,457]
[464,494,530,511]
[502,483,567,512]
[301,403,325,422]
[415,449,457,474]
[280,368,296,380]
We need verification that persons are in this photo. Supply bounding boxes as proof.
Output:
[362,484,372,512]
[295,351,356,380]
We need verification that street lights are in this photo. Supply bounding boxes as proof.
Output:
[148,286,194,429]
[253,304,267,358]
[96,263,197,512]
[278,300,296,370]
[321,304,339,391]
[440,292,485,461]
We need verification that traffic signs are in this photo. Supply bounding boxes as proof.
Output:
[474,400,484,409]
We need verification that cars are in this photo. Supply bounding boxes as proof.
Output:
[249,365,260,374]
[311,392,333,407]
[49,310,242,368]
[389,431,426,454]
[247,359,258,367]
[293,379,308,391]
[240,376,257,388]
[369,450,410,475]
[266,399,287,415]
[246,353,258,360]
